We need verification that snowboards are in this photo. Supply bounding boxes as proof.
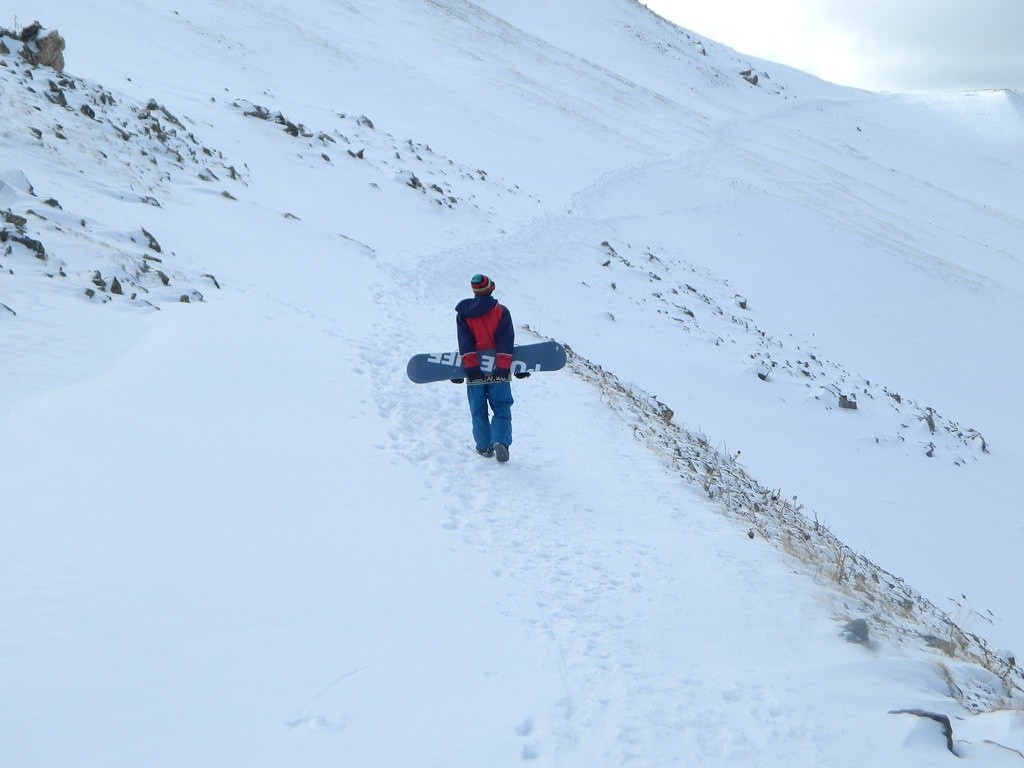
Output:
[405,341,567,386]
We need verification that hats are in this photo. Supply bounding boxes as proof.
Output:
[471,275,495,296]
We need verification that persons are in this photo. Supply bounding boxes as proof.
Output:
[455,274,514,462]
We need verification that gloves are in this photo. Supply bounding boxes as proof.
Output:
[490,367,510,382]
[465,366,490,383]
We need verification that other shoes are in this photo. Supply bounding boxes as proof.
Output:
[478,449,494,458]
[493,443,509,462]
